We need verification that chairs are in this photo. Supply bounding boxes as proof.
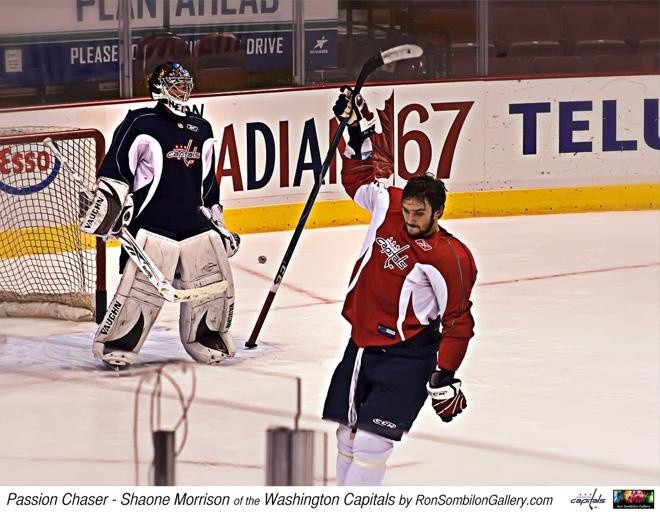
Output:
[509,40,566,74]
[451,43,496,75]
[574,39,627,73]
[638,39,660,71]
[135,29,249,94]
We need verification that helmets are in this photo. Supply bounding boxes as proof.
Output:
[148,61,193,105]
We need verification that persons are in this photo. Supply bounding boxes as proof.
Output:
[76,62,240,368]
[320,84,478,486]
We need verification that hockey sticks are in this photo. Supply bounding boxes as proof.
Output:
[243,45,423,348]
[41,139,229,300]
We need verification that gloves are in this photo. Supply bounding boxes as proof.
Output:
[333,85,376,139]
[427,371,467,422]
